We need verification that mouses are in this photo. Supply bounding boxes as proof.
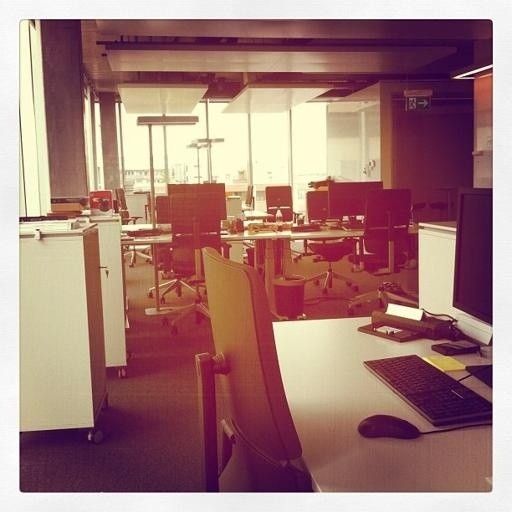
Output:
[358,415,420,440]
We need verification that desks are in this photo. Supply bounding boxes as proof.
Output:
[274,316,493,491]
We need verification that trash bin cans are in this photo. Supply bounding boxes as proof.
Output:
[274,274,306,320]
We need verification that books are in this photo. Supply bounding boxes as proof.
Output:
[19,188,114,236]
[422,354,467,371]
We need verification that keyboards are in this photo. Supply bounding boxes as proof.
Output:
[342,224,365,231]
[363,354,492,425]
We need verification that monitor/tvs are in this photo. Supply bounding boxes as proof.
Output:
[166,183,228,221]
[328,181,383,223]
[451,188,492,389]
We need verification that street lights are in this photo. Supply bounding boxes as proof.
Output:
[188,137,224,184]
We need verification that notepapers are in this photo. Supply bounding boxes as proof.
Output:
[424,356,466,372]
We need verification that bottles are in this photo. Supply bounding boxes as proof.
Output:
[276,209,284,232]
[240,210,247,222]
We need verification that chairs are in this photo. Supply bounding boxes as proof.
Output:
[195,246,311,490]
[112,181,418,336]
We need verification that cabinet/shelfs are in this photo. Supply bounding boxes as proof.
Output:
[418,222,493,344]
[18,213,128,441]
